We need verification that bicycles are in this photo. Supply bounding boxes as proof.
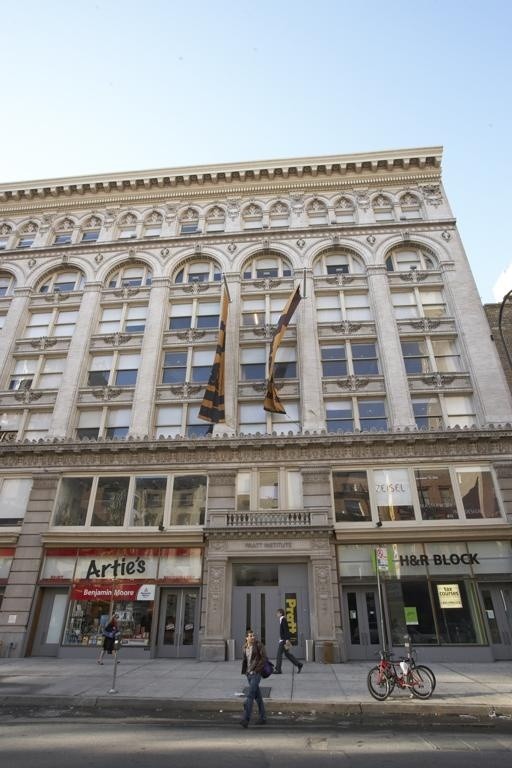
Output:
[366,648,437,701]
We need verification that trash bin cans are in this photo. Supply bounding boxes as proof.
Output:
[227,639,235,660]
[306,639,314,662]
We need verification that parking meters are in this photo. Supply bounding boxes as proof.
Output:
[106,630,123,695]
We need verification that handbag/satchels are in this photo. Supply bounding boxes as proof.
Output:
[103,629,115,639]
[260,656,276,679]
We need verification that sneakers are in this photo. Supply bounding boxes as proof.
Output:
[238,717,267,729]
[97,659,121,665]
[271,663,304,674]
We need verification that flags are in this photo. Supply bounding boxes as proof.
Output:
[195,276,229,426]
[262,282,302,416]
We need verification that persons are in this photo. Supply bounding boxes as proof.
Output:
[272,608,304,675]
[96,613,120,665]
[237,629,268,729]
